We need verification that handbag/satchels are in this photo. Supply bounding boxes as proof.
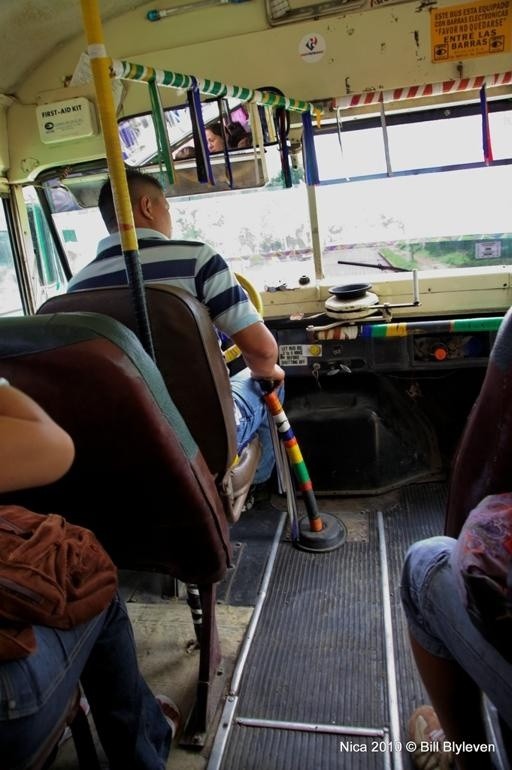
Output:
[0,505,116,659]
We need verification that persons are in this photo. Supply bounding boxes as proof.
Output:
[0,376,181,770]
[174,121,253,160]
[399,488,512,770]
[66,170,285,511]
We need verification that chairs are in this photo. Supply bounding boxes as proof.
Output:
[35,285,263,525]
[444,303,511,536]
[175,107,255,160]
[0,311,233,747]
[5,680,101,770]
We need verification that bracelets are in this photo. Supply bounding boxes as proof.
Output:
[0,377,9,388]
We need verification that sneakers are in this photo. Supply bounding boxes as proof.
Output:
[155,694,179,741]
[407,706,455,769]
[60,698,89,746]
